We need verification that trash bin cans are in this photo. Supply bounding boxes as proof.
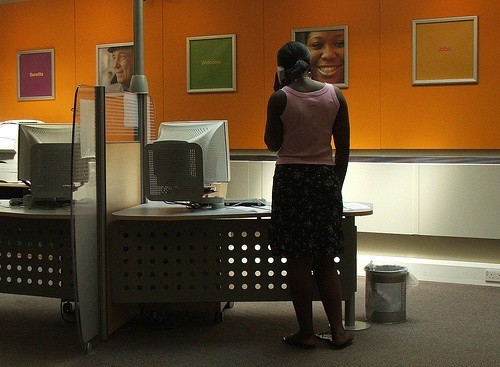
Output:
[365,265,408,324]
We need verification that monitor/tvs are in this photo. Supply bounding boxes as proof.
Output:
[144,119,231,203]
[17,120,89,205]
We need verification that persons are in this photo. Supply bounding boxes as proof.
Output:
[98,46,134,92]
[264,42,354,348]
[305,29,345,83]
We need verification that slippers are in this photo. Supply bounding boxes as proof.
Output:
[330,331,355,350]
[281,333,316,350]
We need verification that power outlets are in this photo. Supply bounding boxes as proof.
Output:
[485,270,500,283]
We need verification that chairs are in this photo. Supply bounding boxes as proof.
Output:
[0,120,45,183]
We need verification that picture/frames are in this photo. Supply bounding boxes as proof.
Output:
[412,16,478,86]
[16,48,55,102]
[95,41,133,96]
[186,34,236,93]
[291,24,349,88]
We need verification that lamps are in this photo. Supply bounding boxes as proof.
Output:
[129,74,149,94]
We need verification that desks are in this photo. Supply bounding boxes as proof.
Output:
[109,204,374,332]
[0,191,98,350]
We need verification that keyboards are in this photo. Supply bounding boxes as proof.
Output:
[9,197,23,206]
[223,197,266,206]
